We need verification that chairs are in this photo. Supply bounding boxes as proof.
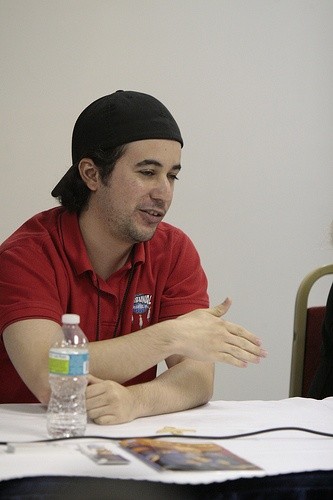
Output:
[288,264,333,400]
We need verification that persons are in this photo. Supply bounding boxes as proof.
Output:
[0,91,267,425]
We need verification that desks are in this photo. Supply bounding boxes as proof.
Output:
[0,403,333,500]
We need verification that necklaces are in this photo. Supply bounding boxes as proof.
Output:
[95,260,134,341]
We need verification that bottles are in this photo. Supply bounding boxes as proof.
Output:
[46,314,89,439]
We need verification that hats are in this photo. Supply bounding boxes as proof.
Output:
[51,90,183,197]
